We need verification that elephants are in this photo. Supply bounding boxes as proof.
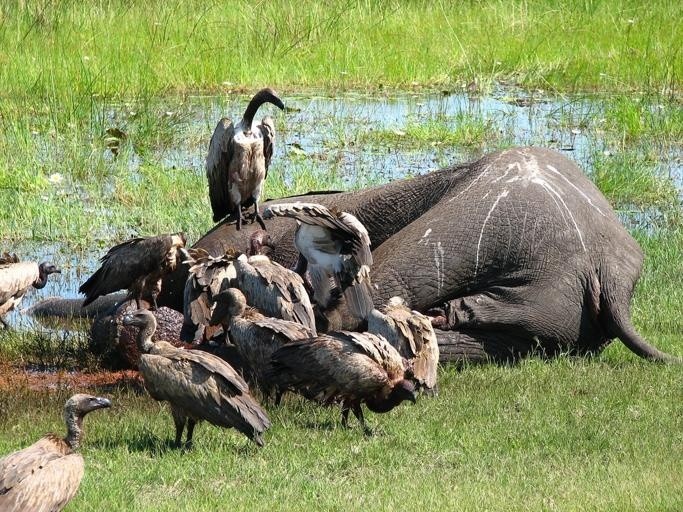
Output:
[25,145,683,367]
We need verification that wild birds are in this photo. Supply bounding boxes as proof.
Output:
[204,85,287,233]
[77,227,190,311]
[0,259,62,331]
[1,393,113,512]
[181,200,442,436]
[121,309,272,451]
